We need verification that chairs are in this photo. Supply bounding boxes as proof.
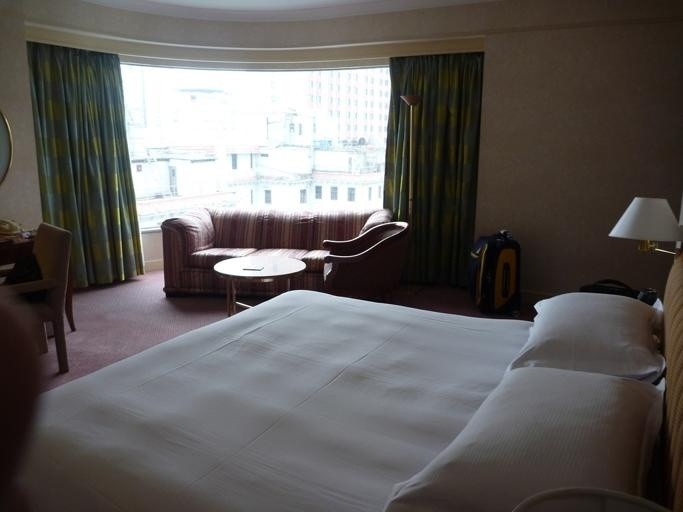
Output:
[321,221,409,298]
[0,222,72,374]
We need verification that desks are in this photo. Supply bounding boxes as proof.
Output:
[0,232,76,332]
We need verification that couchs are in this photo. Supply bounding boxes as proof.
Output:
[161,208,391,297]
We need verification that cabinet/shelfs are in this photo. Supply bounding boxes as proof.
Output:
[0,109,14,183]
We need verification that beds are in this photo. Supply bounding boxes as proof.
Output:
[15,255,682,511]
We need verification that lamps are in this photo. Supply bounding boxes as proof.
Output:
[608,197,683,257]
[399,94,425,296]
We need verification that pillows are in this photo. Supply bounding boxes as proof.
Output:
[2,256,47,303]
[506,293,667,384]
[385,369,665,511]
